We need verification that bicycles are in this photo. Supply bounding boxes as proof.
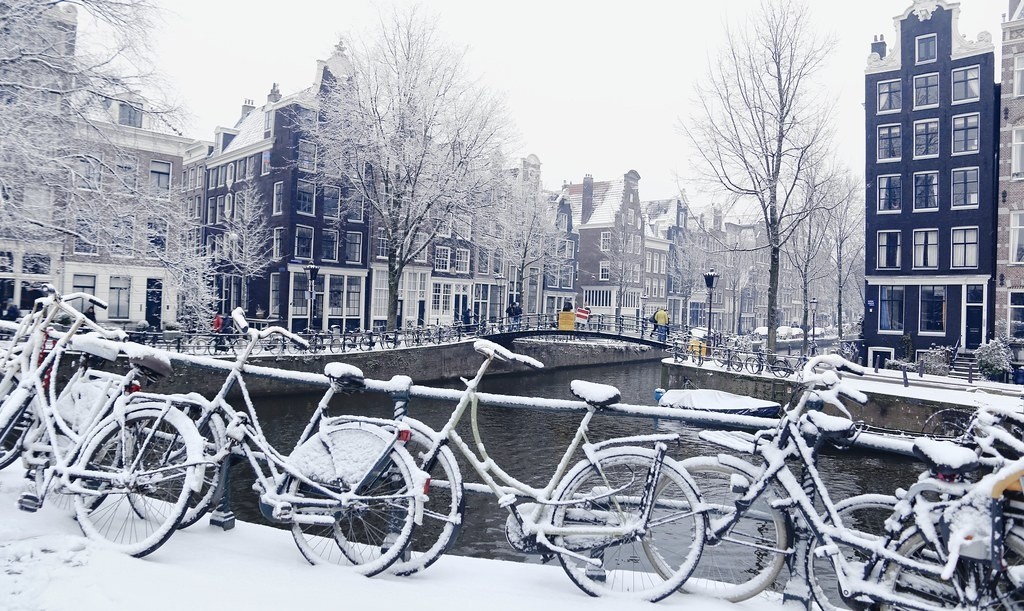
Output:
[0,281,220,560]
[111,308,460,579]
[641,335,1024,611]
[327,339,720,609]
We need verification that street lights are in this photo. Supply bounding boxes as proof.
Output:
[641,294,649,338]
[495,273,506,332]
[302,261,321,340]
[808,297,819,358]
[702,267,721,361]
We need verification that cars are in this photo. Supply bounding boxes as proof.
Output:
[808,327,826,338]
[751,326,804,341]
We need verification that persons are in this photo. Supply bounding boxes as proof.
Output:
[2,298,21,321]
[81,306,96,333]
[563,298,573,312]
[506,302,522,332]
[464,308,472,335]
[576,303,592,330]
[650,308,670,342]
[208,310,223,354]
[214,311,236,356]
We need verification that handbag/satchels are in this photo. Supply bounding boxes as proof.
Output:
[649,315,656,323]
